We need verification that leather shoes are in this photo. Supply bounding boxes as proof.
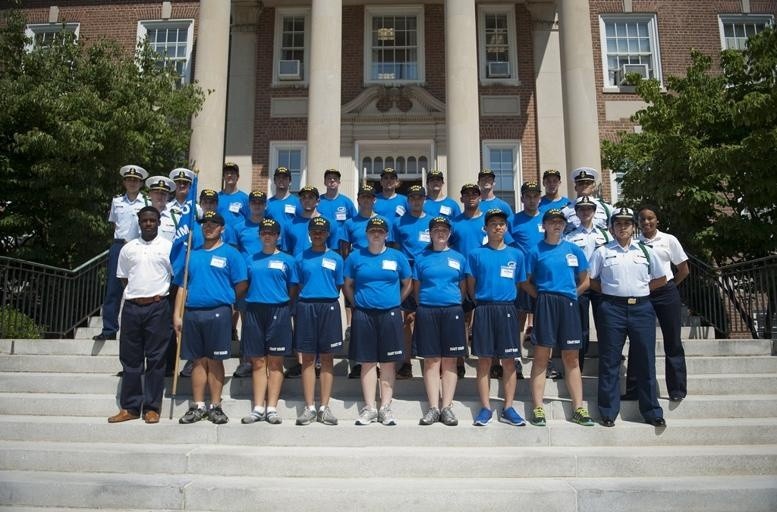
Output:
[93,334,116,340]
[118,367,182,377]
[108,409,160,424]
[601,391,685,425]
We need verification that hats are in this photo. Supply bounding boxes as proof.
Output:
[521,182,542,193]
[380,167,398,181]
[610,207,635,224]
[479,169,496,178]
[542,169,561,180]
[201,211,224,226]
[249,192,266,202]
[259,219,280,235]
[298,185,319,201]
[574,195,597,211]
[407,185,425,197]
[325,169,341,178]
[274,167,291,177]
[428,216,451,231]
[308,217,330,232]
[461,184,481,196]
[365,218,388,233]
[358,185,376,198]
[223,163,239,176]
[485,208,507,225]
[427,171,444,184]
[120,164,195,194]
[200,190,218,205]
[570,166,600,182]
[543,209,567,222]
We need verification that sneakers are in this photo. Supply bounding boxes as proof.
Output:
[530,407,546,426]
[378,408,397,425]
[317,406,338,425]
[355,407,378,425]
[474,408,492,426]
[241,411,266,424]
[420,408,440,425]
[179,407,208,424]
[500,407,526,426]
[571,407,594,426]
[266,412,281,424]
[232,328,561,378]
[181,360,194,377]
[208,405,229,424]
[440,407,458,425]
[296,409,317,425]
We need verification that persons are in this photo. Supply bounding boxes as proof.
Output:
[585,207,668,426]
[136,174,181,247]
[93,165,155,341]
[168,161,617,427]
[106,207,173,423]
[620,208,692,402]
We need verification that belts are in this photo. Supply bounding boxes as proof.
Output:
[129,296,168,306]
[601,294,646,305]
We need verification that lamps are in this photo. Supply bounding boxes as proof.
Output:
[382,80,406,102]
